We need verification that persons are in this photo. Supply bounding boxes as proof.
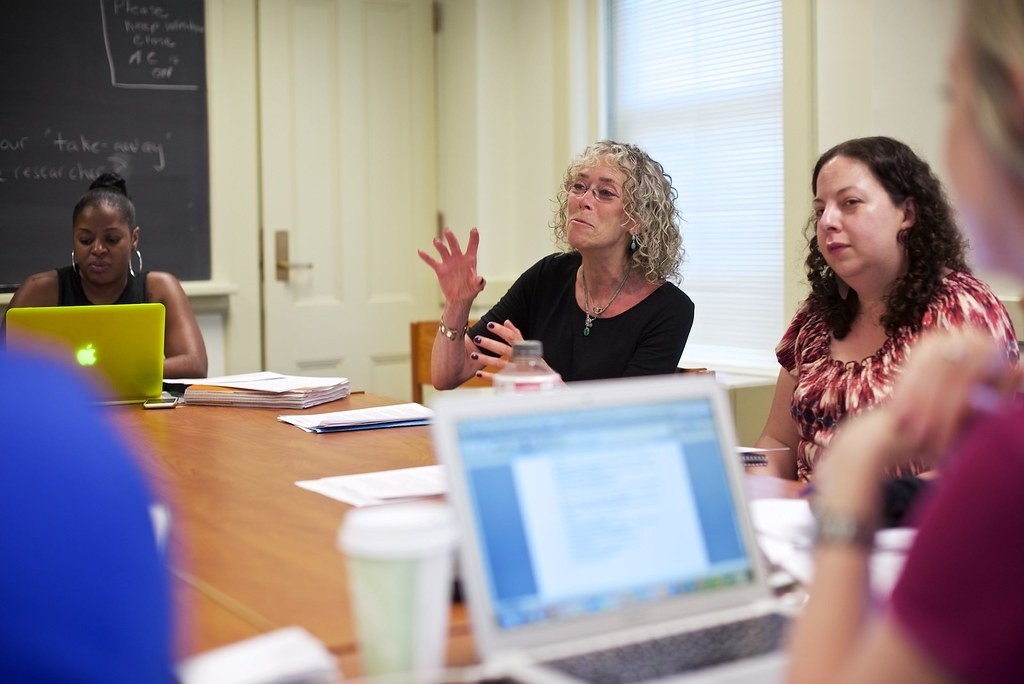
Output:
[789,0,1023,684]
[2,171,208,379]
[418,139,696,391]
[749,137,1020,496]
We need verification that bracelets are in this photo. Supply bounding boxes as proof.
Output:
[817,523,873,550]
[440,317,468,340]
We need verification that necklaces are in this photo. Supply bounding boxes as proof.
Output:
[582,270,629,336]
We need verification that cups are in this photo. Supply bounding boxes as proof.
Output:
[335,500,461,684]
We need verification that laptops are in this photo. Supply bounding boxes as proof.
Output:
[429,374,805,684]
[6,303,166,407]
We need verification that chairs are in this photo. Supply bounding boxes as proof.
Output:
[409,320,511,405]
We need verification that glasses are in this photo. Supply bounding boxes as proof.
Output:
[568,180,626,202]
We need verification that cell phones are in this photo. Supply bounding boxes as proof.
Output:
[144,396,179,409]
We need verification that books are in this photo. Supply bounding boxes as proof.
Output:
[184,377,351,409]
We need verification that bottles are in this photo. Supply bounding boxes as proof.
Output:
[493,340,562,391]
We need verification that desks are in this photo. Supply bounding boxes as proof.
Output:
[100,392,482,684]
